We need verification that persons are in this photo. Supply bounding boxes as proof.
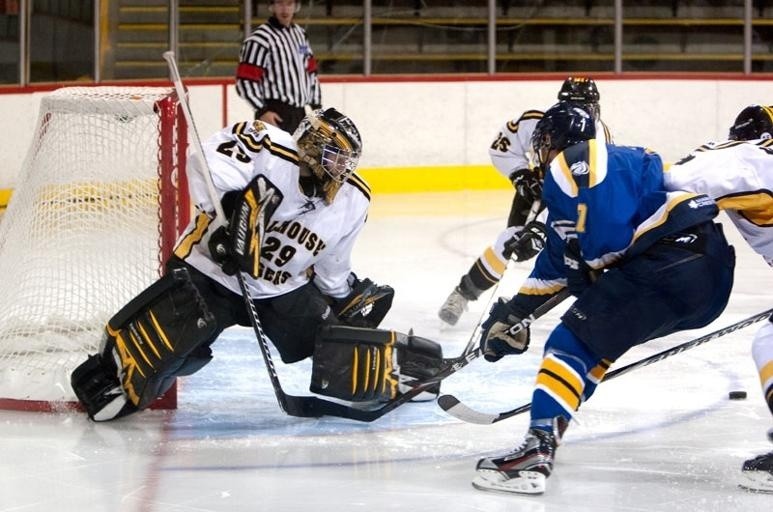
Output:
[439,79,600,326]
[475,104,735,497]
[231,1,321,139]
[663,105,772,492]
[68,108,443,422]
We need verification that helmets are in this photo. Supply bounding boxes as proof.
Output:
[530,103,596,151]
[557,75,601,121]
[297,107,362,203]
[728,105,773,139]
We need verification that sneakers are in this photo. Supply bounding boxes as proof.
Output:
[438,284,477,326]
[744,452,773,471]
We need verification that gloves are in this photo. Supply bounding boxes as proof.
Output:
[503,220,548,261]
[563,237,598,295]
[480,297,530,362]
[509,168,542,199]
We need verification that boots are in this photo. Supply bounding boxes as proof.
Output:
[477,414,569,477]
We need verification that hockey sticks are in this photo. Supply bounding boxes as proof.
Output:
[316,285,572,422]
[164,50,323,418]
[438,310,773,425]
[440,198,542,368]
